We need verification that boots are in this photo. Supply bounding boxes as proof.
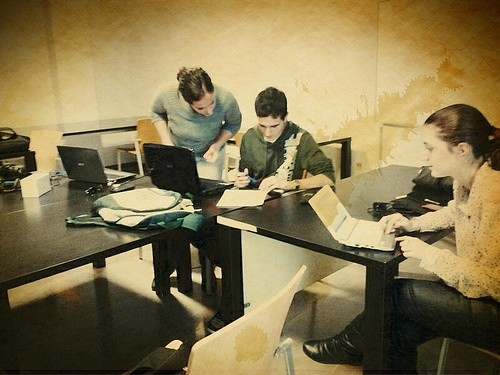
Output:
[304,321,364,365]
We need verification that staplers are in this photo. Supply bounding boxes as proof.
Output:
[110,174,137,192]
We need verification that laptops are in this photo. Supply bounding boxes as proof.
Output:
[141,142,235,199]
[57,145,136,184]
[307,184,398,250]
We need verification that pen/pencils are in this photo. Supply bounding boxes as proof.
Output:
[246,174,258,183]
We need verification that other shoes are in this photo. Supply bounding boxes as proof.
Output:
[152,261,178,290]
[201,269,218,291]
[207,310,235,334]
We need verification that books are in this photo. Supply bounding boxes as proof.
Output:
[216,189,267,208]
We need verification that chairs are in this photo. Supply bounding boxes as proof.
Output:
[118,119,162,176]
[185,265,306,375]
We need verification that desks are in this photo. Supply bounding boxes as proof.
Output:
[0,136,456,375]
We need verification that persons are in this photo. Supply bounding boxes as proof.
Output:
[138,67,243,294]
[206,87,337,337]
[302,104,500,375]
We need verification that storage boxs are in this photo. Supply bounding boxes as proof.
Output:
[20,173,52,197]
